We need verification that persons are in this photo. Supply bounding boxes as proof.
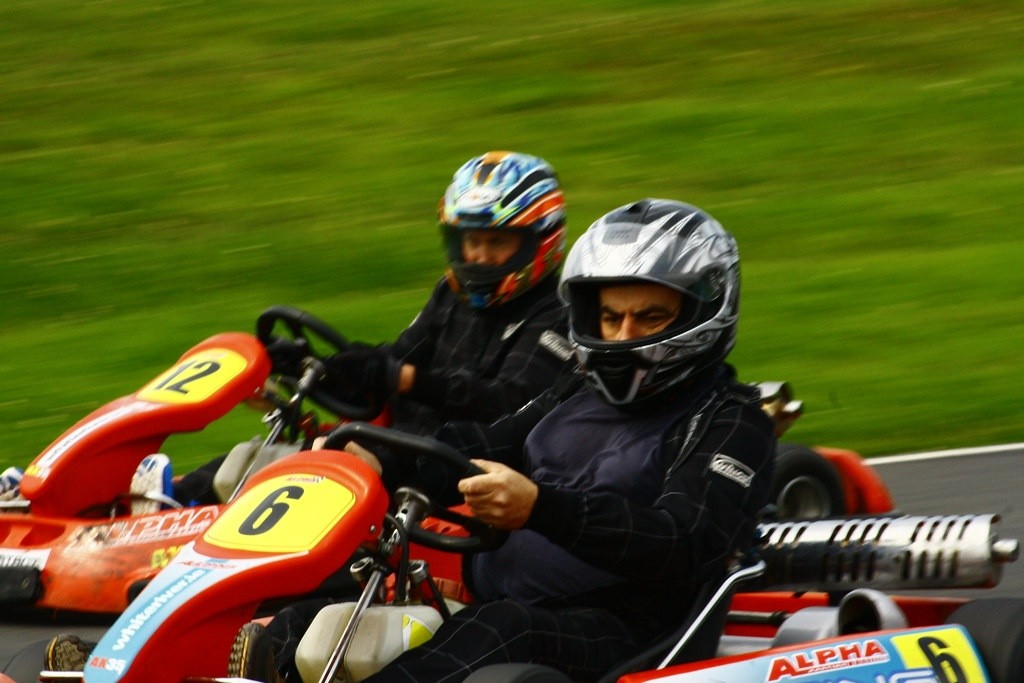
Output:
[43,194,778,683]
[0,151,579,518]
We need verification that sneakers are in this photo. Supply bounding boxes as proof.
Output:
[129,451,174,517]
[0,466,24,495]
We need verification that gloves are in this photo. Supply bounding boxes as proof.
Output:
[320,342,401,405]
[265,332,310,376]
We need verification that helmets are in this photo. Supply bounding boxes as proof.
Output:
[439,148,567,309]
[558,201,742,412]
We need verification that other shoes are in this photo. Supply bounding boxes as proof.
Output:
[44,632,88,672]
[229,621,281,683]
